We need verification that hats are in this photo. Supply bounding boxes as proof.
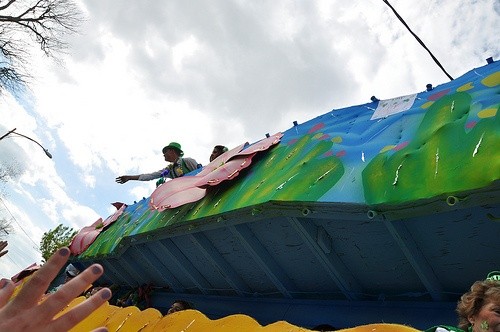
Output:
[162,141,184,156]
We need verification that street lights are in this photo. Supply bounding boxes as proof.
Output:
[0,127,53,160]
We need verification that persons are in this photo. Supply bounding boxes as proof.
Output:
[167,300,189,316]
[115,142,202,184]
[0,241,8,257]
[424,271,500,332]
[210,145,228,163]
[0,247,112,332]
